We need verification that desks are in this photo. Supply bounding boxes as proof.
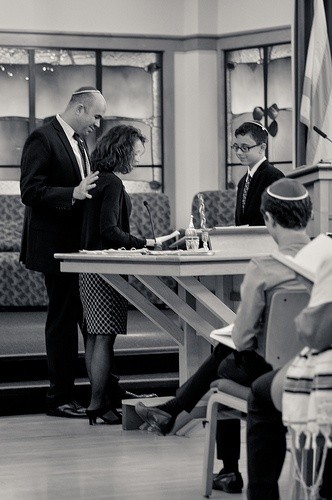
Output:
[56,252,253,437]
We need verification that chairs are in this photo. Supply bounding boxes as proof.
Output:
[201,290,311,495]
[193,191,238,228]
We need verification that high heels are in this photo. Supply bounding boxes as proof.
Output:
[86,405,123,425]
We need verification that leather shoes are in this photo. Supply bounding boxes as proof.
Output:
[47,400,88,417]
[116,391,158,408]
[213,472,243,493]
[135,401,176,435]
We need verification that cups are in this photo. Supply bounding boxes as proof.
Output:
[185,237,199,251]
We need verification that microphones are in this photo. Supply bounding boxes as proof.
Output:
[144,201,163,250]
[314,126,332,143]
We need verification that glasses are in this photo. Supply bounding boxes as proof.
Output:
[233,141,264,152]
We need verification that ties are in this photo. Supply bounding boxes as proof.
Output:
[74,132,87,179]
[242,174,251,210]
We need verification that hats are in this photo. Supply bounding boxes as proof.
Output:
[267,179,309,201]
[245,120,269,135]
[73,87,99,95]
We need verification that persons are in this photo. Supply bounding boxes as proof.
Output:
[231,120,286,226]
[134,178,312,494]
[246,252,332,500]
[78,124,163,426]
[19,86,108,419]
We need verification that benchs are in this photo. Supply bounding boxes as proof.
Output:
[0,193,172,313]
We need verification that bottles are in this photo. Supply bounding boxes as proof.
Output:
[185,215,197,237]
